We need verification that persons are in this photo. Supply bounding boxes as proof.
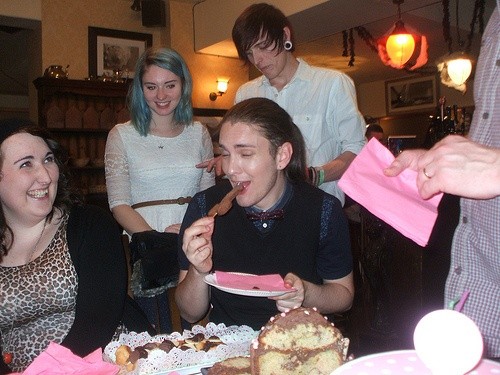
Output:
[365,124,384,145]
[174,97,354,331]
[195,3,368,208]
[0,118,157,375]
[383,0,500,362]
[105,45,216,243]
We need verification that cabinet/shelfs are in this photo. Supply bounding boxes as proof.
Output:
[33,75,130,208]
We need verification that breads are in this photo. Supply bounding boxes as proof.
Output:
[115,334,226,372]
[206,355,253,375]
[248,306,347,375]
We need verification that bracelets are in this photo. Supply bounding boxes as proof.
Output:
[305,166,324,188]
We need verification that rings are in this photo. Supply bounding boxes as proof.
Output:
[423,168,432,179]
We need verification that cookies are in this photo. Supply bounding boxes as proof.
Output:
[208,184,244,216]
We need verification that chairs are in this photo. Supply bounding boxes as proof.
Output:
[355,203,427,334]
[122,232,197,332]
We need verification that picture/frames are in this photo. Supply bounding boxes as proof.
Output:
[87,25,153,83]
[384,71,439,116]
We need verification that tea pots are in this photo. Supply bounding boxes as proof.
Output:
[44,64,70,80]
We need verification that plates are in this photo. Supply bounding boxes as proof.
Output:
[202,272,297,297]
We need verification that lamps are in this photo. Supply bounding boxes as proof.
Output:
[435,0,484,86]
[386,0,415,66]
[210,77,230,100]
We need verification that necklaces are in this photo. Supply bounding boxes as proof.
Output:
[149,124,176,149]
[0,220,47,364]
